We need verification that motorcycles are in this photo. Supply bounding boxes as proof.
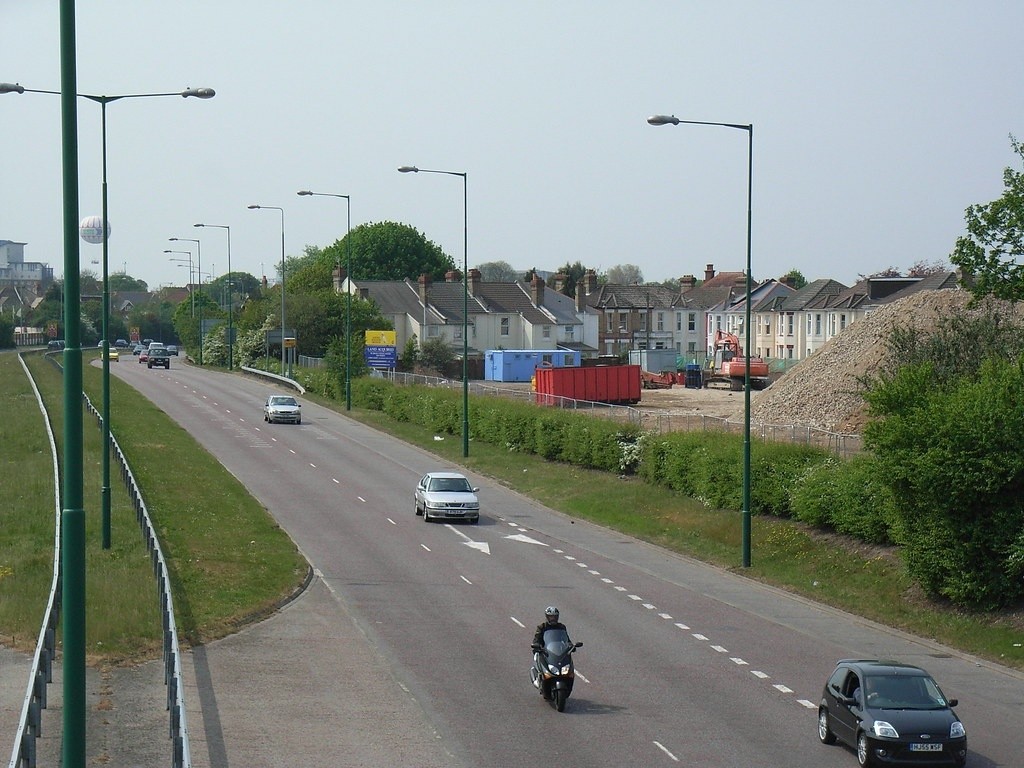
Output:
[531,628,583,713]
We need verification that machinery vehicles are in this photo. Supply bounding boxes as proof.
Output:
[703,328,771,392]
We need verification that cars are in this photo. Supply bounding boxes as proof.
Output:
[100,347,119,362]
[819,658,967,768]
[97,340,113,349]
[115,338,129,348]
[133,345,146,355]
[129,341,142,348]
[414,472,480,524]
[166,345,178,356]
[263,395,302,425]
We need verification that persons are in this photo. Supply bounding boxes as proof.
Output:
[531,606,576,654]
[852,677,880,705]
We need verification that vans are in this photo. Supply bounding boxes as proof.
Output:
[149,342,163,353]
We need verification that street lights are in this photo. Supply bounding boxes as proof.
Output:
[246,204,286,378]
[644,113,754,572]
[396,164,470,459]
[168,237,202,366]
[0,80,215,549]
[295,189,352,413]
[194,223,233,371]
[162,250,211,320]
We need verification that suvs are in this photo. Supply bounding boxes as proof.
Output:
[142,338,154,346]
[48,340,65,349]
[147,349,172,369]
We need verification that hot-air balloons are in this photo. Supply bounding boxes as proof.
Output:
[80,216,112,265]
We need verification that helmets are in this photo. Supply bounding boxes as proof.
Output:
[545,606,560,626]
